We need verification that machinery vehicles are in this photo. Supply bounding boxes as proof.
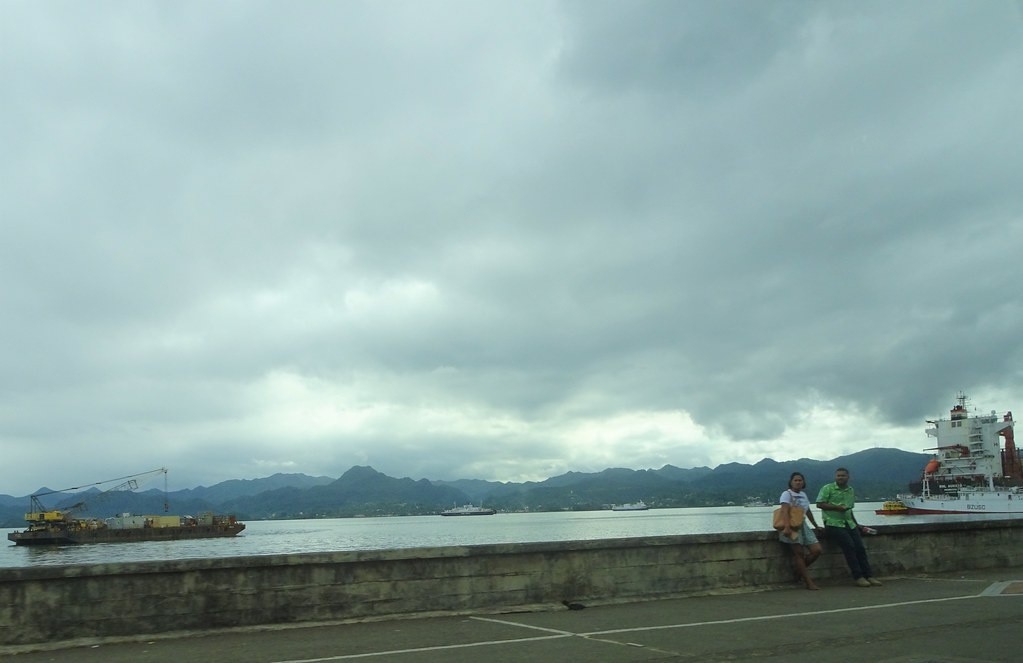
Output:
[24,467,170,532]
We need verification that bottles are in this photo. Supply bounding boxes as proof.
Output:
[861,526,876,535]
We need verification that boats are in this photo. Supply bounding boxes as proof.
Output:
[873,391,1023,516]
[6,523,245,547]
[440,500,497,516]
[612,498,648,511]
[924,460,938,474]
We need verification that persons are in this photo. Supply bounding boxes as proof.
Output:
[778,472,823,590]
[816,468,882,586]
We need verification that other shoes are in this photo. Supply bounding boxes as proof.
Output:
[794,561,802,581]
[855,577,871,587]
[807,584,820,589]
[866,576,882,587]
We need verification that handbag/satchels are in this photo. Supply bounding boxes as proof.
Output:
[773,491,803,532]
